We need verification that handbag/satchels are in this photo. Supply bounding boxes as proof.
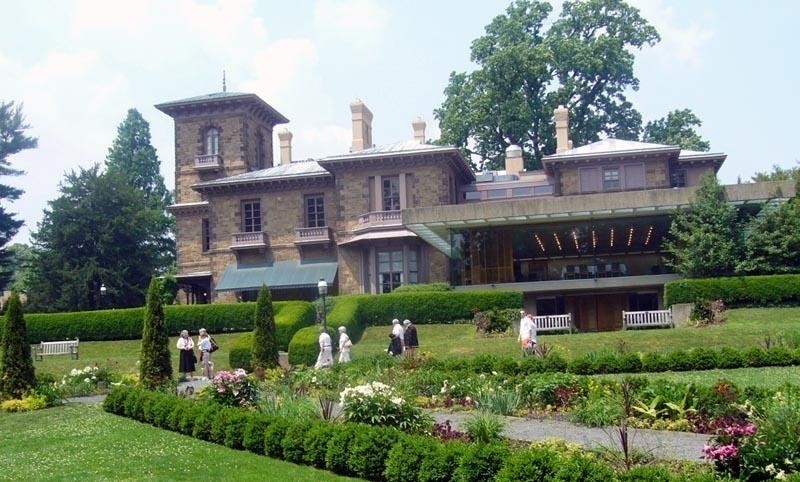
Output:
[192,353,197,363]
[210,339,219,353]
[342,339,352,346]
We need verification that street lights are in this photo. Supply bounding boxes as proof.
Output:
[318,278,328,333]
[101,284,108,308]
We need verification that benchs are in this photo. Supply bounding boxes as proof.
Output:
[530,312,573,335]
[33,335,80,360]
[621,307,674,331]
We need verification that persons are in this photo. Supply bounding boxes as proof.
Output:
[337,325,352,363]
[195,334,212,352]
[197,327,213,379]
[177,329,196,377]
[387,318,404,355]
[315,327,334,369]
[387,333,403,357]
[517,310,537,353]
[527,312,534,319]
[402,319,419,356]
